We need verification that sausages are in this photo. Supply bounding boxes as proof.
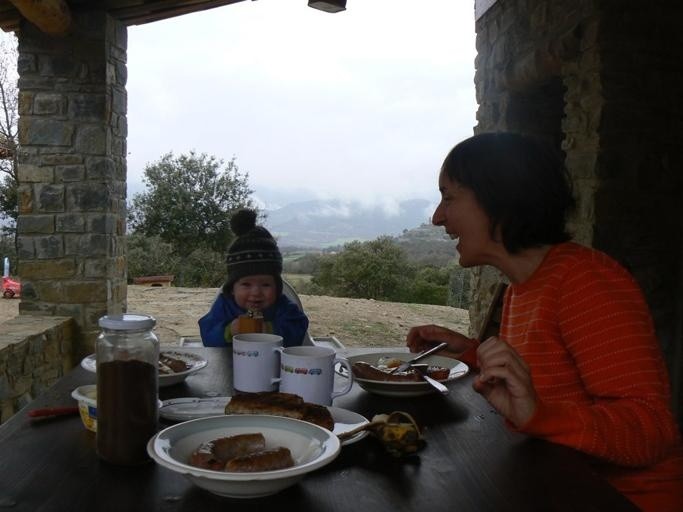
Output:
[350,361,450,383]
[224,447,289,473]
[190,433,264,472]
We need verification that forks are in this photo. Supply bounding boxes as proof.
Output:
[412,367,451,395]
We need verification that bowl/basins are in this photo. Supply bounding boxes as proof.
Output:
[334,352,469,398]
[70,385,162,444]
[146,413,342,499]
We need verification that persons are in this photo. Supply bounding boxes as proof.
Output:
[407,134,683,512]
[198,207,309,347]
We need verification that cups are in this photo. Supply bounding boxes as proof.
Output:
[279,344,353,411]
[230,332,285,393]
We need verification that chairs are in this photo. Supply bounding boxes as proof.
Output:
[216,278,315,345]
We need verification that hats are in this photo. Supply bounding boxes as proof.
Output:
[223,208,282,298]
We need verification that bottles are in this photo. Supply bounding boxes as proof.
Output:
[96,309,160,462]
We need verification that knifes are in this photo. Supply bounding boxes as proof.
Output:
[387,342,448,373]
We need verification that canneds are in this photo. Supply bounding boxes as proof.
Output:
[95,314,160,472]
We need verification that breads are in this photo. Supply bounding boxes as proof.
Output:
[225,392,334,432]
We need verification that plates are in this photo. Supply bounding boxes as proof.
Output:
[162,398,371,450]
[78,349,206,389]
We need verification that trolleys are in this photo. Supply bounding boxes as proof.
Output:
[1,258,20,299]
